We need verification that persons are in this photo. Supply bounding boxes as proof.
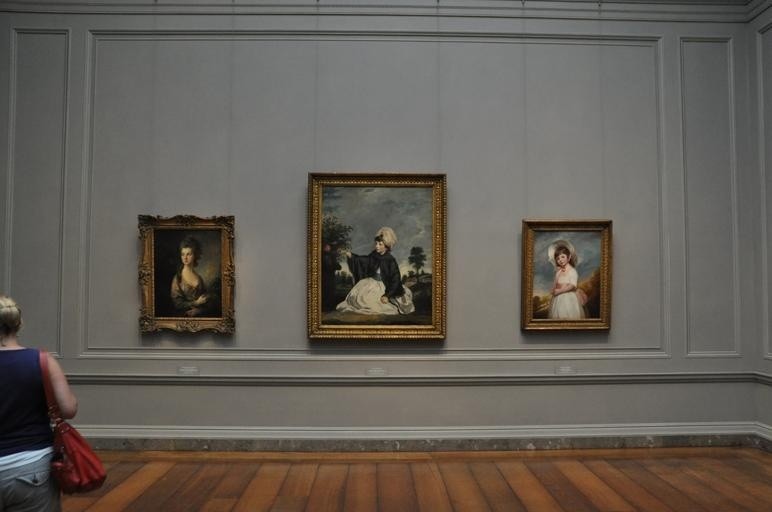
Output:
[545,238,590,318]
[168,235,213,318]
[0,291,82,512]
[336,225,417,315]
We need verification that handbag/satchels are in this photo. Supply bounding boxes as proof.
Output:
[46,417,107,496]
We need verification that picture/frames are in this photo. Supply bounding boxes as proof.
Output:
[304,169,450,347]
[133,211,237,340]
[518,217,616,334]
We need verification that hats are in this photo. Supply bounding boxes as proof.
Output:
[547,238,577,269]
